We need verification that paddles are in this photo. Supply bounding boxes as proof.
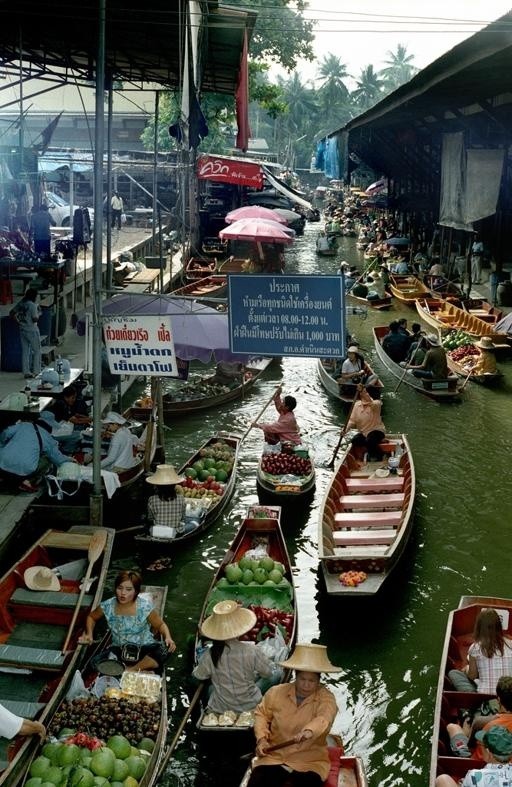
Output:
[61,529,108,654]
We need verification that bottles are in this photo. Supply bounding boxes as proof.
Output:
[24,384,32,403]
[59,369,64,385]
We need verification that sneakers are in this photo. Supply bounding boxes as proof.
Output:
[19,482,38,493]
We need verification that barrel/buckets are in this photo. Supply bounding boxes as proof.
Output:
[455,256,466,276]
[489,271,512,307]
[54,358,71,383]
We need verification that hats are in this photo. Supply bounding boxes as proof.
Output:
[423,334,441,347]
[378,263,389,272]
[102,412,126,425]
[369,469,400,478]
[347,346,359,354]
[201,600,258,641]
[276,644,343,675]
[23,566,61,592]
[146,465,184,486]
[340,261,349,267]
[40,410,62,429]
[369,269,380,279]
[474,725,512,756]
[476,337,495,349]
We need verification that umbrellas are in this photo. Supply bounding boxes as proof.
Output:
[224,205,289,227]
[218,218,292,259]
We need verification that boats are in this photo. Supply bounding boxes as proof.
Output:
[0,524,116,786]
[318,211,409,275]
[444,298,504,324]
[316,327,385,408]
[21,583,169,786]
[193,504,298,732]
[415,296,509,339]
[429,595,512,787]
[317,430,415,596]
[422,274,465,300]
[45,406,157,520]
[134,355,273,415]
[256,442,316,495]
[387,273,433,305]
[239,753,367,786]
[372,325,459,397]
[134,430,242,544]
[344,286,395,309]
[436,324,504,383]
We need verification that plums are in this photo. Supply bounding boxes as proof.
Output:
[47,695,162,746]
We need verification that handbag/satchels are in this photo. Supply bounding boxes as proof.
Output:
[120,642,141,662]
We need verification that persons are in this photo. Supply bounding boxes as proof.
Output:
[319,182,443,305]
[251,387,301,446]
[333,334,378,384]
[1,701,47,747]
[10,287,43,379]
[77,569,178,674]
[0,410,81,488]
[470,232,484,285]
[49,384,90,428]
[26,203,57,256]
[245,669,338,786]
[111,190,124,230]
[470,336,498,375]
[380,318,448,379]
[143,464,198,535]
[88,411,134,473]
[447,673,512,759]
[190,598,276,712]
[448,607,511,695]
[339,381,385,460]
[432,724,512,786]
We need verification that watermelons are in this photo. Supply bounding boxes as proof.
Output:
[216,557,284,586]
[185,457,232,480]
[24,736,155,787]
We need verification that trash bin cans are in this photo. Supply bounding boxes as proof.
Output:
[55,239,78,276]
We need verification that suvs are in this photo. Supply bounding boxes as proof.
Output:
[1,190,95,237]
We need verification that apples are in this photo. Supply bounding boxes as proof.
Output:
[239,604,293,645]
[448,344,480,361]
[180,476,223,496]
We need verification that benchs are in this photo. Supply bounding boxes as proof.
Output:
[333,457,412,560]
[1,589,96,718]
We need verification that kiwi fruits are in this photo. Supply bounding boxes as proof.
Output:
[174,484,221,509]
